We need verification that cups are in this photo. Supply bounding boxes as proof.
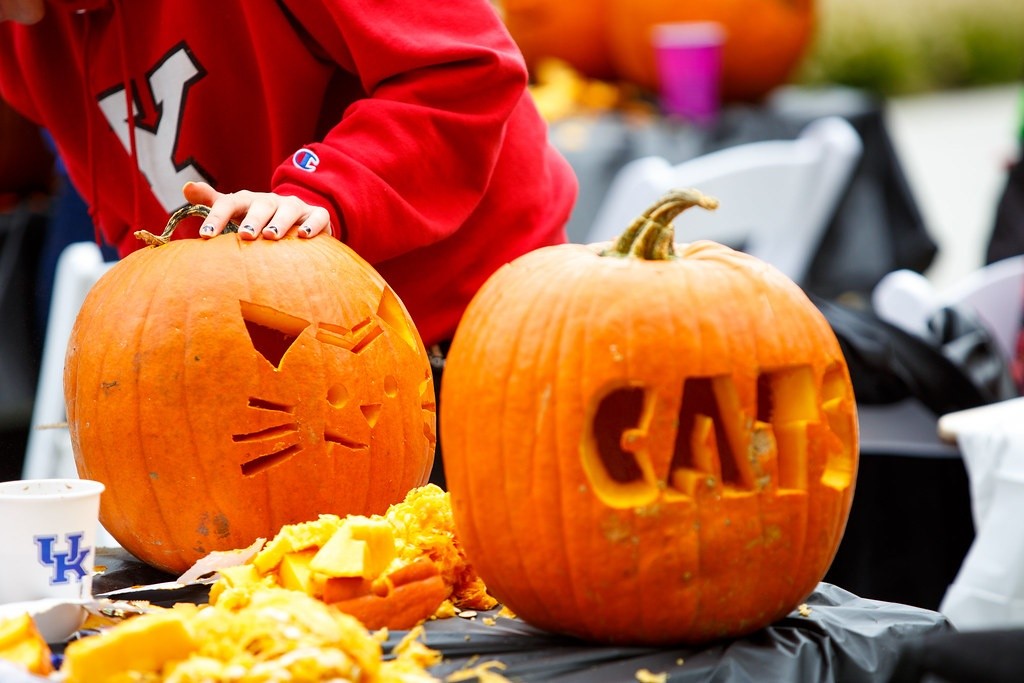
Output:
[651,20,725,126]
[0,478,106,606]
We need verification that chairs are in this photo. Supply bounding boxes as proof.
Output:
[591,112,863,283]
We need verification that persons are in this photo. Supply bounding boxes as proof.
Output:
[0,1,576,495]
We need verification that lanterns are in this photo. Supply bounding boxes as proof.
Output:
[56,226,439,573]
[436,238,864,649]
